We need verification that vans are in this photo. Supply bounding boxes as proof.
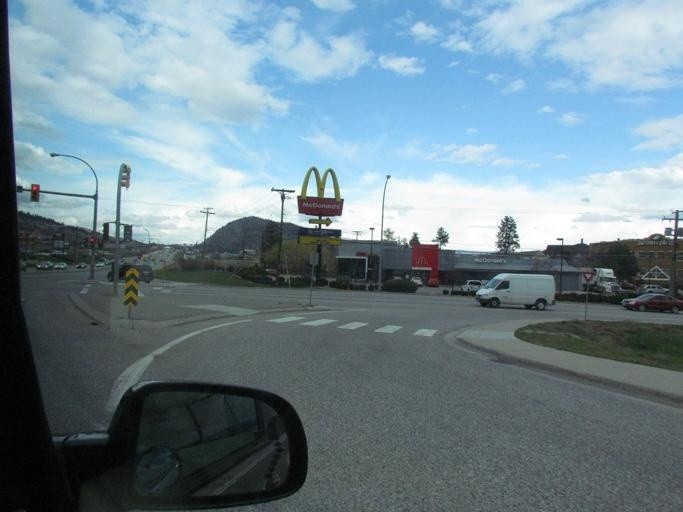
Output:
[475,273,556,311]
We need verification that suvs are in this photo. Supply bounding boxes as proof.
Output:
[106,264,153,283]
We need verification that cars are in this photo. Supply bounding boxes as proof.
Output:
[136,391,289,499]
[75,262,87,268]
[182,245,195,255]
[619,281,682,313]
[18,260,26,272]
[95,257,129,266]
[54,262,68,270]
[410,277,423,287]
[35,260,53,271]
[460,279,481,292]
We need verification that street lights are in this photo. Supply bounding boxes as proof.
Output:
[377,174,391,289]
[49,153,98,281]
[369,227,375,262]
[556,237,563,295]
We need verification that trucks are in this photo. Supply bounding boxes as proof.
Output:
[239,250,256,260]
[581,267,621,293]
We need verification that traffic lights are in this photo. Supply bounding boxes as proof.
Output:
[30,184,39,202]
[97,239,104,249]
[89,236,95,245]
[120,165,131,189]
[102,223,109,240]
[123,224,131,242]
